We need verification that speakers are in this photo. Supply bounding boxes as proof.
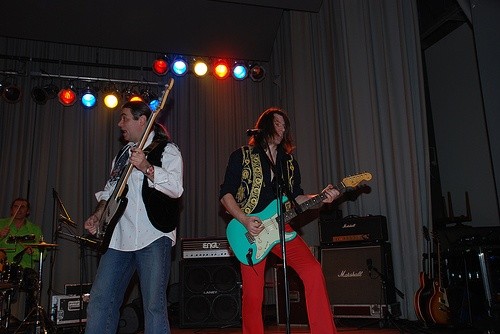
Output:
[177,259,242,330]
[320,244,397,329]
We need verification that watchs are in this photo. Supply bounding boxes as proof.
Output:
[143,164,154,176]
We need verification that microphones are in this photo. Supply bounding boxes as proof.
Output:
[246,129,262,137]
[57,214,77,228]
[366,259,373,275]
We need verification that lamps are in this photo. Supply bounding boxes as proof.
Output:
[0,75,23,103]
[30,53,266,112]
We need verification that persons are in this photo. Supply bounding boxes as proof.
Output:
[218,109,339,334]
[84,102,185,334]
[0,197,47,321]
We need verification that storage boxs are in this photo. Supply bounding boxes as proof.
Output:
[52,294,87,324]
[274,263,308,326]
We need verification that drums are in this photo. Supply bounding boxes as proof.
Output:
[0,250,39,291]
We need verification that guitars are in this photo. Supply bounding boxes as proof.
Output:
[97,76,176,254]
[414,225,453,326]
[225,169,372,266]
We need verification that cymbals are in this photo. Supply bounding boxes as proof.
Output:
[23,242,63,249]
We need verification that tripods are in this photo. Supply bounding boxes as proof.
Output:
[13,246,58,334]
